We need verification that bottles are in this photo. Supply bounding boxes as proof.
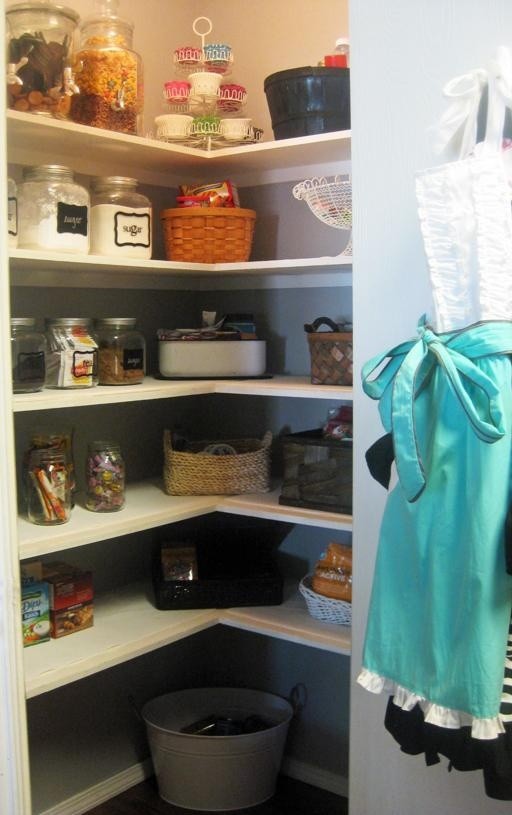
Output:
[94,318,147,387]
[87,175,153,260]
[73,0,145,139]
[10,317,47,394]
[24,450,70,525]
[7,166,91,255]
[85,442,126,512]
[3,1,80,122]
[43,318,100,389]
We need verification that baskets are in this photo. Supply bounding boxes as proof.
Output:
[299,574,352,626]
[161,428,273,496]
[292,173,352,231]
[159,207,258,263]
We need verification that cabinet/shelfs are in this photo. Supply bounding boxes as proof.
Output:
[2,0,355,814]
